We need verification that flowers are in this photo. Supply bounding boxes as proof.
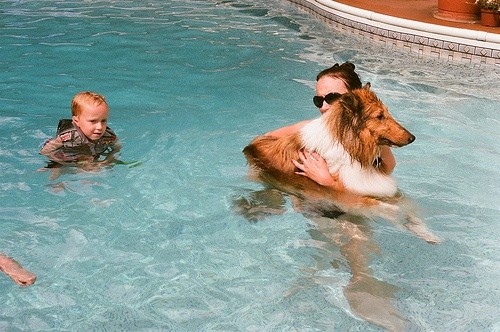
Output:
[473,0,500,11]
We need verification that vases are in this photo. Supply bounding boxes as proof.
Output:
[480,8,500,27]
[438,0,479,23]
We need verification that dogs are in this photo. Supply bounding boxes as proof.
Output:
[239,82,440,245]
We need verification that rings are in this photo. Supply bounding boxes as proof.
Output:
[301,158,305,162]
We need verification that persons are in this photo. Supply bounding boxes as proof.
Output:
[0,250,38,287]
[233,60,397,286]
[36,90,123,193]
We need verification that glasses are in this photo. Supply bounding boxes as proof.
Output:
[313,93,342,108]
